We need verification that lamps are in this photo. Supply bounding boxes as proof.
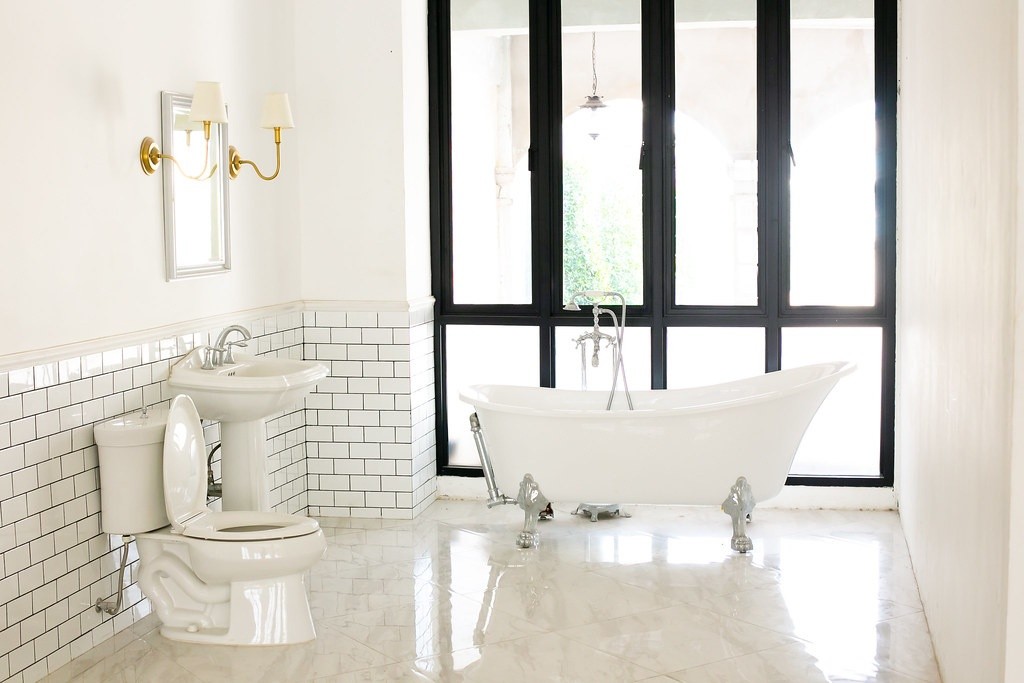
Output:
[579,33,608,140]
[226,91,294,181]
[139,79,228,188]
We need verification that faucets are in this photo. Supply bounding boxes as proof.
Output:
[212,324,252,367]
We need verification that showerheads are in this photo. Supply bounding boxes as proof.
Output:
[563,290,615,311]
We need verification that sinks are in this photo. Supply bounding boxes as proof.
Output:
[167,345,332,426]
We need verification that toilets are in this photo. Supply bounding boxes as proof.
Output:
[90,392,327,650]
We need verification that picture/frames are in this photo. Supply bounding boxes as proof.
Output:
[159,88,232,284]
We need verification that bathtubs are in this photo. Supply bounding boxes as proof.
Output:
[459,360,862,555]
